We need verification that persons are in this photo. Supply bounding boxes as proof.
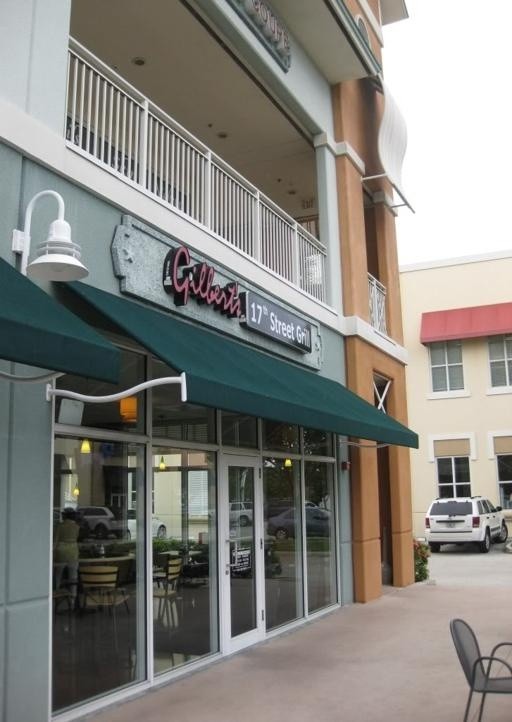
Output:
[53,507,81,614]
[75,508,91,544]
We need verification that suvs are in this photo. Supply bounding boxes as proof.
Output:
[426,494,510,552]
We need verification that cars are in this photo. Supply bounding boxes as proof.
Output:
[54,504,167,540]
[207,500,331,540]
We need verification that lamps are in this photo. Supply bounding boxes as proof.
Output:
[120,395,138,423]
[80,439,90,454]
[10,188,89,281]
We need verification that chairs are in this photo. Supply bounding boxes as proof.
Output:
[50,518,246,690]
[449,618,511,722]
